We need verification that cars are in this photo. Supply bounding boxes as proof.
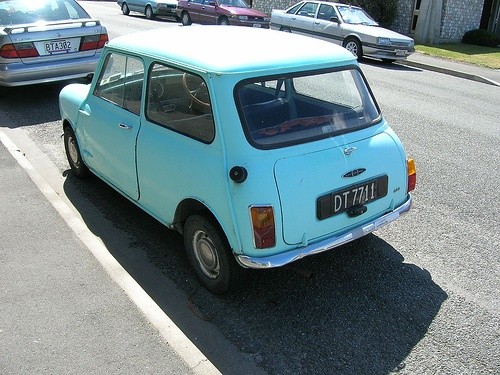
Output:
[175,0,268,29]
[59,24,417,298]
[117,0,179,20]
[0,0,110,86]
[268,0,415,63]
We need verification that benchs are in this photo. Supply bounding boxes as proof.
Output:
[166,98,288,139]
